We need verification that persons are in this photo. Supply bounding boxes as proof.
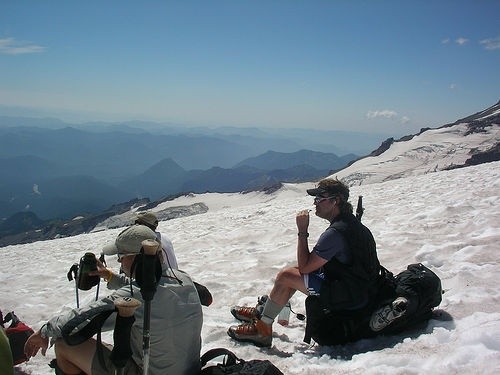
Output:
[228,178,374,347]
[135,211,178,270]
[0,327,15,375]
[23,223,203,375]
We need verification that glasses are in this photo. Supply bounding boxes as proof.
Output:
[117,253,139,261]
[315,197,333,204]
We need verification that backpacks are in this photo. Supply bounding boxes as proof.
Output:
[192,348,284,375]
[304,263,444,346]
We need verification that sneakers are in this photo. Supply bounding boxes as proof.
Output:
[231,306,260,322]
[227,319,273,348]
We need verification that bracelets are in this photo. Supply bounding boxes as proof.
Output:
[298,232,309,237]
[104,270,112,282]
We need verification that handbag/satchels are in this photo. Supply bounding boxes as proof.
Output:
[3,311,35,365]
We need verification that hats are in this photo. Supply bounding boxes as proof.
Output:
[135,213,158,227]
[102,224,162,255]
[306,179,350,198]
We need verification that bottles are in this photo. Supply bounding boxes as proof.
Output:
[77,252,98,291]
[277,300,306,327]
